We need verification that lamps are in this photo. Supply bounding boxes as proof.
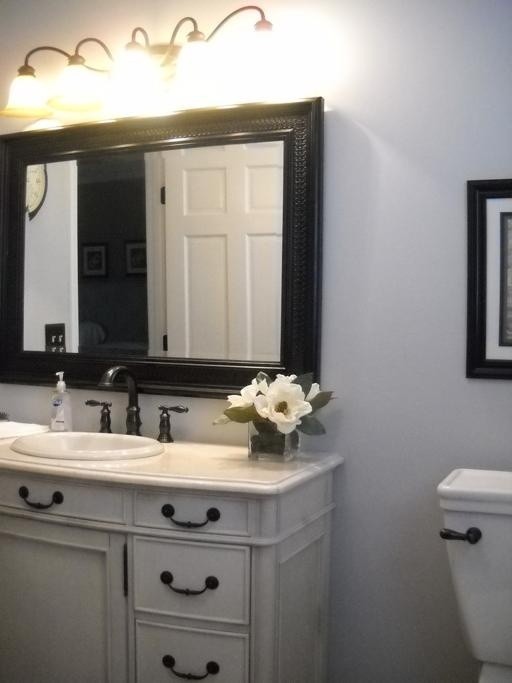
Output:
[2,6,273,123]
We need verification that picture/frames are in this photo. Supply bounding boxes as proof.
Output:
[124,240,146,274]
[465,179,512,379]
[80,242,108,278]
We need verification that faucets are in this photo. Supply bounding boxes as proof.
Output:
[98,366,143,436]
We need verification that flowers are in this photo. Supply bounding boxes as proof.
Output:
[215,370,338,454]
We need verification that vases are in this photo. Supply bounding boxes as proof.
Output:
[248,419,300,463]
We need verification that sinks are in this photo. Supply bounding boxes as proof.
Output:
[10,431,164,461]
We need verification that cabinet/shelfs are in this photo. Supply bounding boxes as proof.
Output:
[0,417,346,683]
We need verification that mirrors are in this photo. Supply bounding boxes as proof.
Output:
[1,97,324,398]
